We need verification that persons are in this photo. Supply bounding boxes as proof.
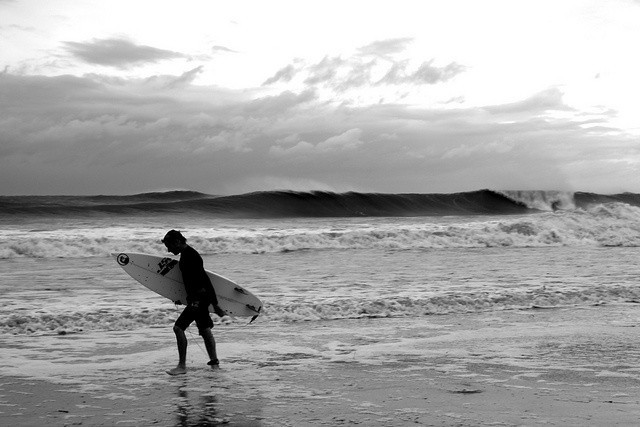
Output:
[161,229,225,375]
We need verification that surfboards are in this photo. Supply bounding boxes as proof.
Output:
[111,252,263,317]
